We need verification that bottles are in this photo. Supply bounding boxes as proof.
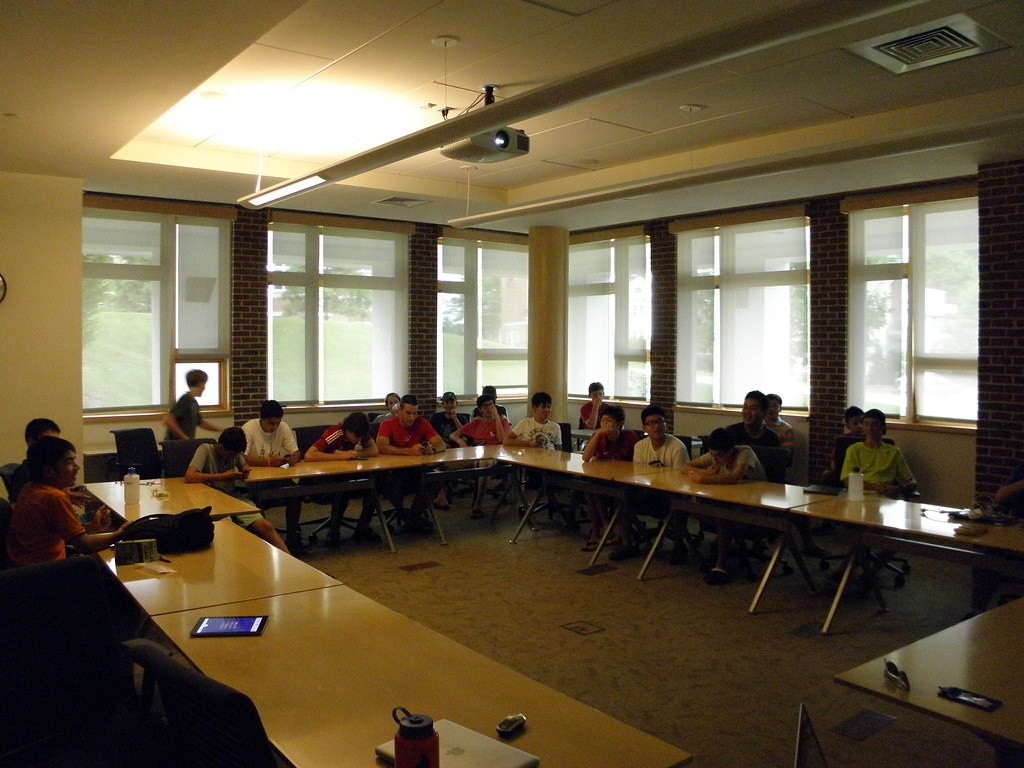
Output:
[124,467,140,505]
[392,404,399,410]
[848,467,864,503]
[392,706,440,768]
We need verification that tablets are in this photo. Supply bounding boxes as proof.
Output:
[189,616,269,637]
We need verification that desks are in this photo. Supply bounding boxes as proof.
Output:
[81,443,1024,768]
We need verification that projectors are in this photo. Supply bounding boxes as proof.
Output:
[440,127,529,164]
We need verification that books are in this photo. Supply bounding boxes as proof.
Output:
[804,483,843,495]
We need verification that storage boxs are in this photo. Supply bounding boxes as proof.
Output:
[114,539,159,567]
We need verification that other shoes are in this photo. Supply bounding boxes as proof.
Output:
[703,569,731,585]
[286,537,303,555]
[353,527,383,540]
[608,544,642,561]
[326,530,340,544]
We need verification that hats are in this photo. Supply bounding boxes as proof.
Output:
[441,392,457,400]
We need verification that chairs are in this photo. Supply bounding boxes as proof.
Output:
[641,436,704,555]
[0,463,20,503]
[0,553,279,768]
[369,422,412,532]
[518,423,589,531]
[159,438,218,478]
[819,436,921,602]
[434,411,473,495]
[158,450,164,478]
[577,417,586,452]
[0,497,20,570]
[83,453,121,483]
[473,406,507,500]
[368,412,383,422]
[292,425,361,545]
[110,427,162,479]
[631,429,647,551]
[701,446,794,582]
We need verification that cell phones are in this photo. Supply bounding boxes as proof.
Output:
[356,456,369,460]
[422,440,428,447]
[496,713,527,736]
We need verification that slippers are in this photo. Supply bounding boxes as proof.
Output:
[582,541,602,552]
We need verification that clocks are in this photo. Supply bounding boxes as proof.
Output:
[0,274,7,304]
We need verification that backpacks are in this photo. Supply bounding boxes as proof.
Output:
[121,506,214,552]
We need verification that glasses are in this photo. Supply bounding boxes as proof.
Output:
[481,403,495,409]
[884,657,910,690]
[645,418,667,426]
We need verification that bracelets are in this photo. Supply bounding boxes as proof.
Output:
[268,457,271,467]
[430,444,437,454]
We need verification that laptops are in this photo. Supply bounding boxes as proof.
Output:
[375,719,540,768]
[947,509,1019,527]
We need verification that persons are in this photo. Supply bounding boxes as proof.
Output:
[503,391,588,518]
[428,393,469,449]
[763,392,796,468]
[580,405,639,552]
[238,400,315,554]
[369,393,446,535]
[679,428,769,585]
[303,413,382,545]
[726,390,830,559]
[186,426,292,556]
[449,386,508,520]
[164,369,224,441]
[5,435,148,644]
[608,404,691,565]
[960,462,1024,624]
[821,406,865,482]
[840,411,918,500]
[580,382,608,450]
[10,419,93,507]
